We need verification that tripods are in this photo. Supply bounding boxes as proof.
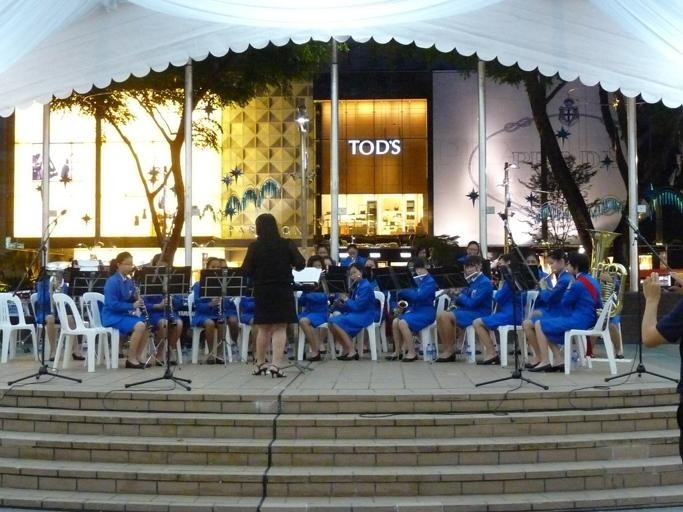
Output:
[279,289,315,376]
[124,253,196,392]
[474,256,549,389]
[604,242,680,386]
[6,248,84,385]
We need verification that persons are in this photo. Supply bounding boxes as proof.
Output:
[526,252,623,373]
[102,252,183,367]
[193,257,268,363]
[36,268,86,361]
[642,272,683,462]
[386,241,526,365]
[298,245,380,361]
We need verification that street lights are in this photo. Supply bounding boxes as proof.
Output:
[500,161,519,263]
[293,105,314,263]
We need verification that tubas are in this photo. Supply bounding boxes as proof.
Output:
[584,228,626,316]
[45,260,71,315]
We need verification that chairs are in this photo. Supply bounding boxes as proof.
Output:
[232,296,254,361]
[0,293,38,363]
[177,342,183,365]
[498,291,538,367]
[564,293,618,374]
[29,292,60,361]
[420,295,450,357]
[81,290,125,368]
[293,291,338,359]
[461,290,498,362]
[53,291,111,373]
[188,290,235,362]
[330,292,388,360]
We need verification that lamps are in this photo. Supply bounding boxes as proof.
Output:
[497,160,519,253]
[292,105,311,259]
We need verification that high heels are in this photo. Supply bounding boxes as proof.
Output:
[617,354,624,359]
[252,362,267,376]
[270,364,287,378]
[72,354,85,360]
[50,358,54,360]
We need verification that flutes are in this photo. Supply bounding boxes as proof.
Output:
[412,273,426,279]
[328,274,361,314]
[535,269,558,291]
[437,271,477,301]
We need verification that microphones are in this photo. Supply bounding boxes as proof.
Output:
[43,207,70,226]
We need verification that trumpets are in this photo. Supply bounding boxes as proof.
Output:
[395,300,408,317]
